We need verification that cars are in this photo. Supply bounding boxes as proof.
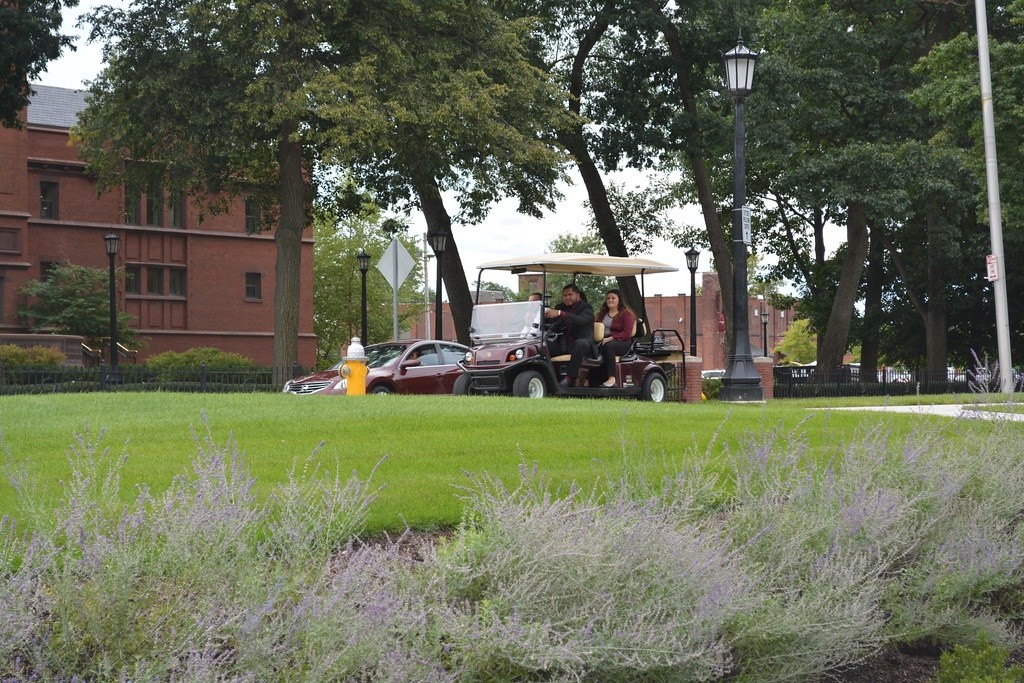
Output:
[848,363,911,383]
[948,366,992,382]
[280,339,476,398]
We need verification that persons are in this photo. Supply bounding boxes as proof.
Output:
[596,289,635,387]
[407,351,427,366]
[519,292,543,337]
[545,284,595,386]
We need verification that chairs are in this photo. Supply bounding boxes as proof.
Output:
[615,320,637,363]
[637,317,666,356]
[551,322,605,366]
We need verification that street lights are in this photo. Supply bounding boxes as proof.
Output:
[712,29,766,405]
[759,309,770,357]
[356,250,373,349]
[683,246,701,360]
[102,225,122,370]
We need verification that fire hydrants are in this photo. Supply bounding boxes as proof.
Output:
[337,336,370,395]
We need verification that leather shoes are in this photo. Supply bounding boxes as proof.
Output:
[597,382,617,388]
[557,376,577,387]
